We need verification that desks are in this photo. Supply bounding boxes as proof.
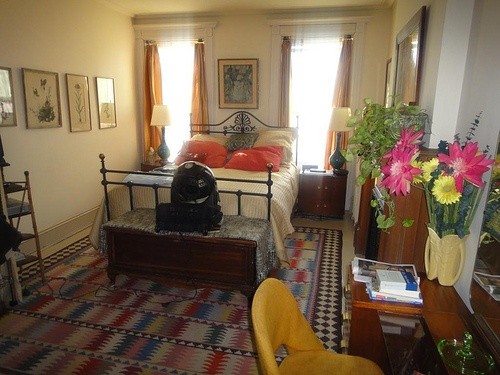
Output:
[359,148,446,265]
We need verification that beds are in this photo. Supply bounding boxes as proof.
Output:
[89,111,297,272]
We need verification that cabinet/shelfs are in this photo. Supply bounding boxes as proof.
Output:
[340,263,500,375]
[0,165,44,301]
[102,208,271,304]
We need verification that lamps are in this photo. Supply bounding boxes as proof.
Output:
[326,105,352,173]
[150,104,173,161]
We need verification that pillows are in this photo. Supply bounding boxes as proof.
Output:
[176,128,295,173]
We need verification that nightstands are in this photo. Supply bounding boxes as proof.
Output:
[300,172,347,219]
[142,161,161,172]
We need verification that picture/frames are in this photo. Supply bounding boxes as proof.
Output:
[95,77,118,129]
[0,67,17,127]
[218,58,258,109]
[23,67,62,129]
[65,73,92,133]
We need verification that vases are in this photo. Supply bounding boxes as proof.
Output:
[424,226,463,286]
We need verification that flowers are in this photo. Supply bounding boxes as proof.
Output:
[381,124,496,238]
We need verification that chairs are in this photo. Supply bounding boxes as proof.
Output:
[251,279,386,375]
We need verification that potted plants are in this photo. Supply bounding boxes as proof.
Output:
[343,96,432,232]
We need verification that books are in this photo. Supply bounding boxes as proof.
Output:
[366,269,423,305]
